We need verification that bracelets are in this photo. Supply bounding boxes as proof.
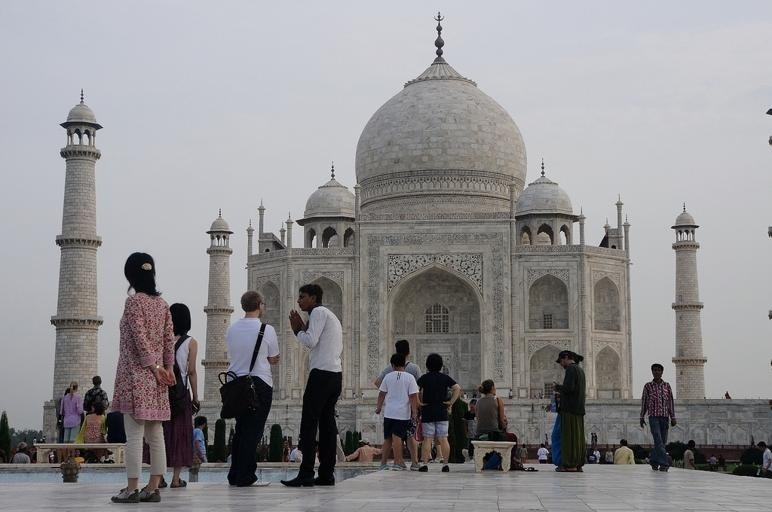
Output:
[152,364,160,373]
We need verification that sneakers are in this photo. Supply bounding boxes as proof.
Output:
[399,464,407,470]
[139,486,161,502]
[379,464,389,472]
[441,465,449,472]
[391,464,401,471]
[410,463,420,471]
[158,478,168,488]
[170,479,187,488]
[111,489,141,503]
[418,466,428,472]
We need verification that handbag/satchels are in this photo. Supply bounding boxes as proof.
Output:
[218,371,258,420]
[411,417,424,442]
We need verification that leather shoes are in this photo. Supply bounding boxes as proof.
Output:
[281,474,315,487]
[237,479,271,487]
[312,475,335,486]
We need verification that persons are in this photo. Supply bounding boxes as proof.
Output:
[725,390,731,399]
[374,339,551,472]
[346,437,382,462]
[110,251,178,504]
[550,348,587,473]
[639,363,677,471]
[276,282,344,487]
[282,439,319,463]
[718,454,724,471]
[756,440,772,479]
[709,454,716,470]
[54,375,127,464]
[336,428,346,463]
[222,289,281,488]
[142,301,202,488]
[188,415,207,482]
[683,439,697,470]
[587,432,636,464]
[8,435,47,464]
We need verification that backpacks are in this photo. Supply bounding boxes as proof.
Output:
[168,335,191,418]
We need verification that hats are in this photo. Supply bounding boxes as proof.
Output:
[555,350,584,364]
[359,438,370,443]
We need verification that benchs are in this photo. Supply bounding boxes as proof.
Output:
[32,442,127,463]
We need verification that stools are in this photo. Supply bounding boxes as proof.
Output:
[471,439,517,472]
